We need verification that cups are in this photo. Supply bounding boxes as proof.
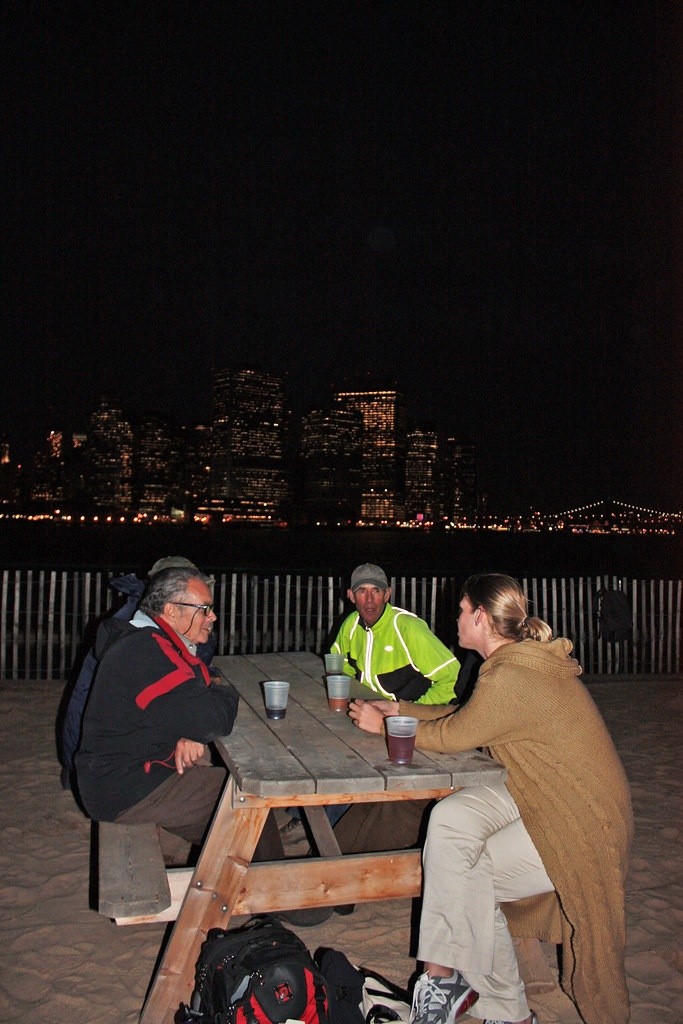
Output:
[326,676,352,713]
[386,716,419,764]
[263,681,290,719]
[324,654,344,675]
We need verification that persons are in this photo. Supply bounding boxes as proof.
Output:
[278,564,460,844]
[63,557,333,925]
[347,574,635,1024]
[306,652,487,912]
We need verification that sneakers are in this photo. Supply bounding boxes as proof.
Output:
[483,1008,538,1024]
[409,968,479,1024]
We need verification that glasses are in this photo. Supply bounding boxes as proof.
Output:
[170,602,214,616]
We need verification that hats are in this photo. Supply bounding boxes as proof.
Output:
[148,557,216,585]
[351,562,388,593]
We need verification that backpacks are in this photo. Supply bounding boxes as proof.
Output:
[592,587,633,642]
[174,913,331,1024]
[314,946,411,1024]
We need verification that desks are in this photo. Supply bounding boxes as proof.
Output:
[140,651,505,1024]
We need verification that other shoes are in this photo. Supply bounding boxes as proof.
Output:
[280,818,300,832]
[334,904,355,915]
[264,907,333,927]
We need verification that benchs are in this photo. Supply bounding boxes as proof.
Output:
[99,821,172,918]
